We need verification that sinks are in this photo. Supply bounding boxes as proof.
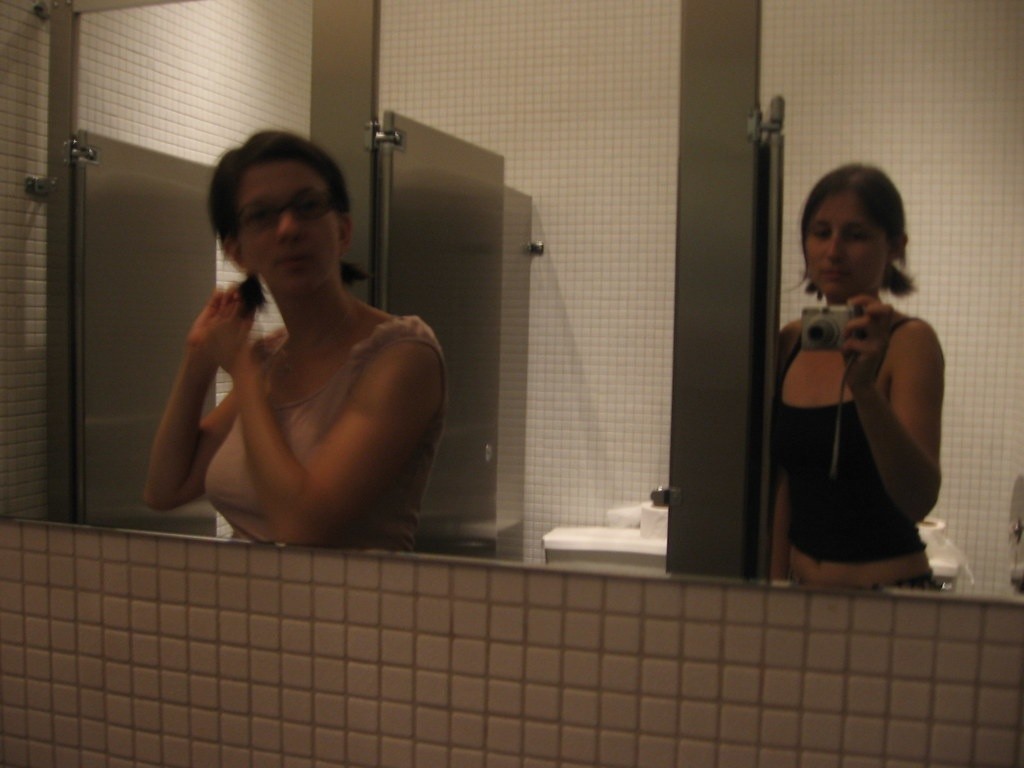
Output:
[541,525,667,570]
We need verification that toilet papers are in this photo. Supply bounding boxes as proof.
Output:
[640,500,669,540]
[915,516,947,559]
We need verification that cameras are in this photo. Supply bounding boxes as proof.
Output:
[802,307,850,350]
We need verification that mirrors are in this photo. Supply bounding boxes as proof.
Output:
[0,0,1024,608]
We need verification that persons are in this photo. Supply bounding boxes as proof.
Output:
[770,165,946,588]
[147,131,448,552]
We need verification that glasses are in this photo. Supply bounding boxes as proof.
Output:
[234,188,335,233]
